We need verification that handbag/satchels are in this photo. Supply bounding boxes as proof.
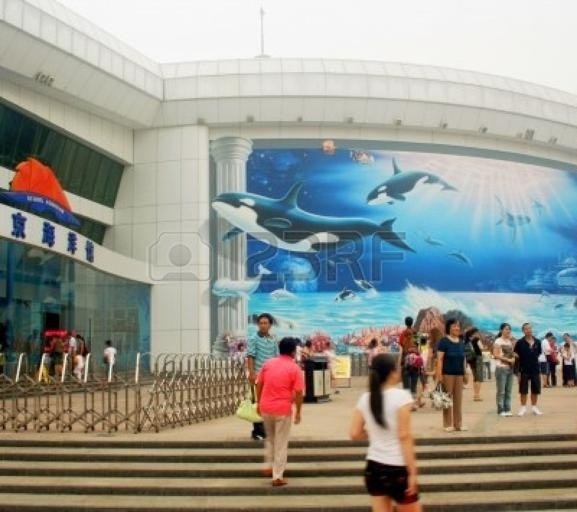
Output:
[431,383,452,409]
[236,384,264,423]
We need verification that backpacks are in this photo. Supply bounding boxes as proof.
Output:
[465,337,477,362]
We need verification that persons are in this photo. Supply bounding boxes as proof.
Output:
[102,340,117,383]
[232,315,576,432]
[350,353,421,512]
[23,327,90,382]
[255,336,304,485]
[245,313,280,441]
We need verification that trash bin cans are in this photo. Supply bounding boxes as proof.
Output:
[303,356,333,403]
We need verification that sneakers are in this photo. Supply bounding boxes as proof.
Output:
[273,480,288,485]
[518,407,528,417]
[531,408,543,416]
